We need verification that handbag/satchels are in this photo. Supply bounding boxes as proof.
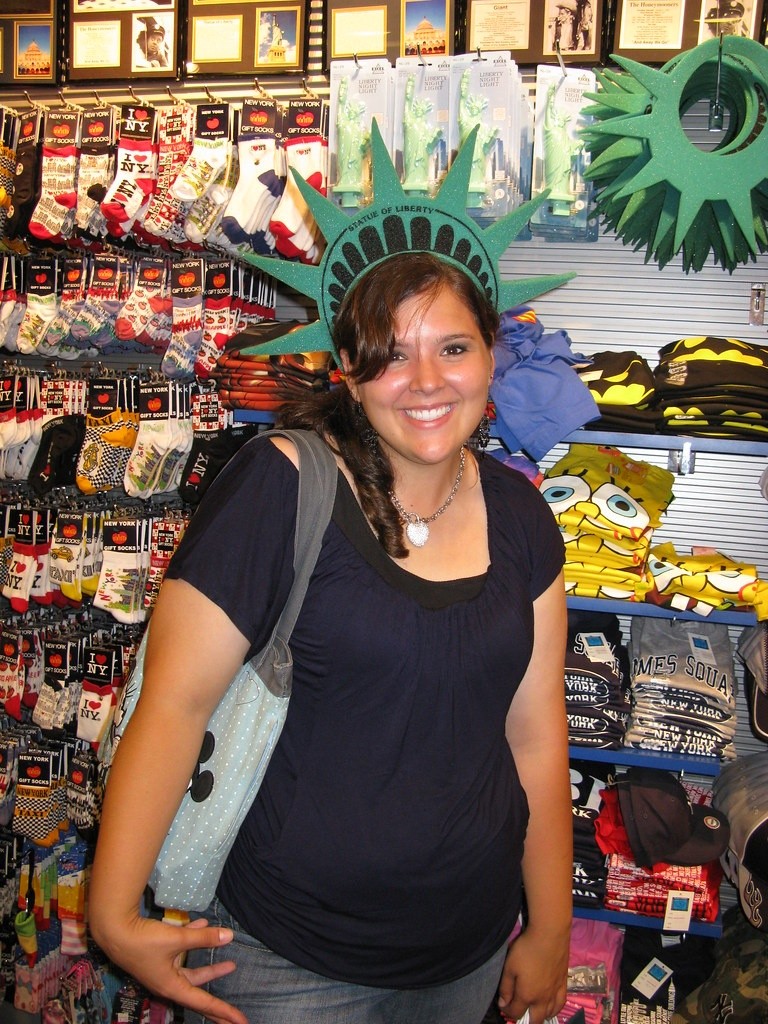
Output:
[96,431,337,912]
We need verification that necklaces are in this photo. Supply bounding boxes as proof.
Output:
[385,449,466,548]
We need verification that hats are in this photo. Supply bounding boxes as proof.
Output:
[594,770,731,871]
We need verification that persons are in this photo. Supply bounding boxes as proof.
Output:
[88,252,574,1024]
